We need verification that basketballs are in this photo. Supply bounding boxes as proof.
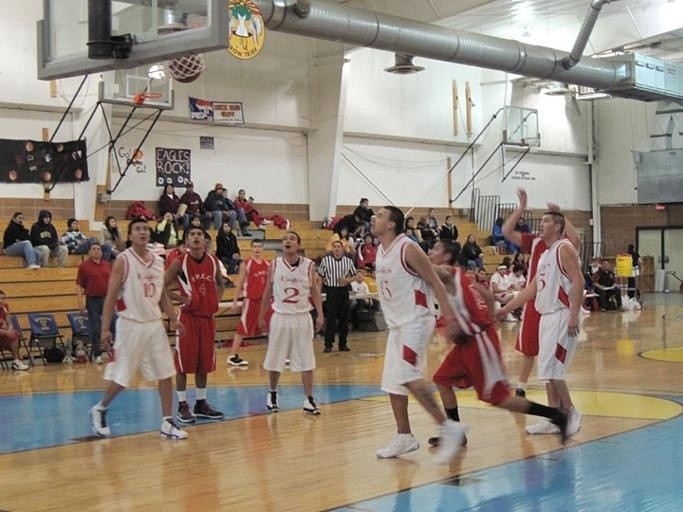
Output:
[170,54,202,81]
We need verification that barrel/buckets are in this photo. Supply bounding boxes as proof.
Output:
[617,341,632,357]
[615,254,633,274]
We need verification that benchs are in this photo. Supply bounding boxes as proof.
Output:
[1,193,646,373]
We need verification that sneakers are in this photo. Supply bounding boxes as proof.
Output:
[226,354,249,366]
[88,401,111,440]
[324,346,332,353]
[193,399,224,420]
[303,394,321,416]
[264,390,279,412]
[566,409,583,438]
[554,408,570,446]
[176,403,197,424]
[525,418,559,435]
[376,433,419,459]
[339,345,351,352]
[428,435,469,447]
[159,421,188,441]
[514,390,526,399]
[94,356,103,364]
[437,419,468,465]
[11,359,29,371]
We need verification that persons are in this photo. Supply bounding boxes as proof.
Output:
[367,206,471,465]
[158,225,231,424]
[422,240,571,446]
[500,186,581,398]
[256,226,322,416]
[499,209,586,439]
[88,214,190,442]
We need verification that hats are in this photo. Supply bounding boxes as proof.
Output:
[214,183,224,191]
[185,182,194,187]
